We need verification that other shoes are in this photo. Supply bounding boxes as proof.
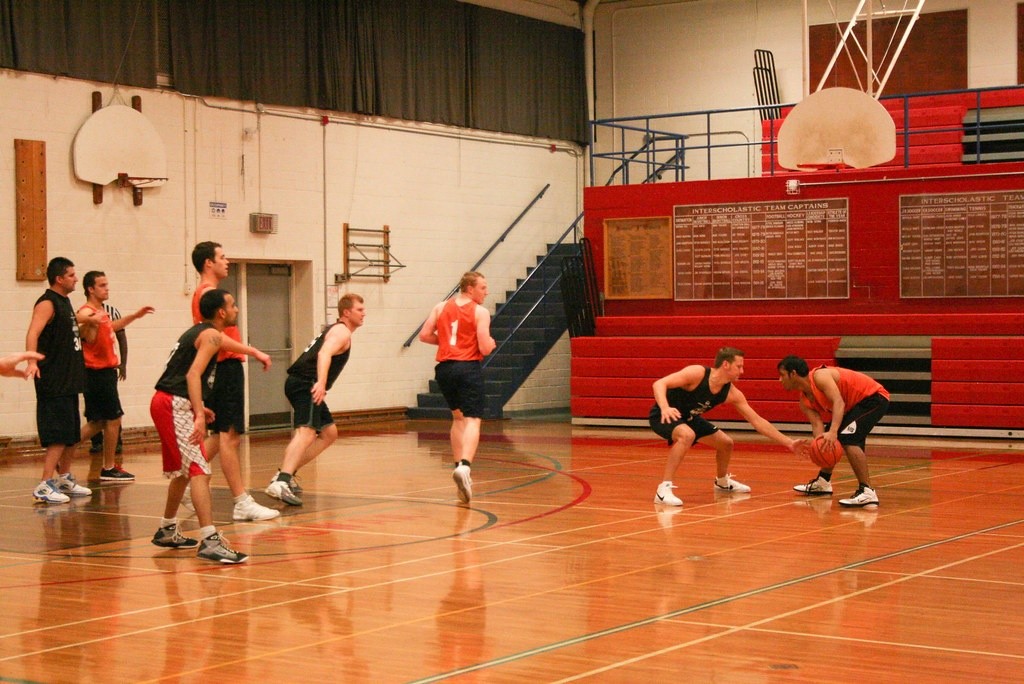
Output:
[452,460,474,504]
[90,442,103,453]
[116,444,121,452]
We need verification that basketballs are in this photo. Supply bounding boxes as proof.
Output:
[809,435,843,469]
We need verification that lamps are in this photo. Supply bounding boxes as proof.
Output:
[786,179,800,196]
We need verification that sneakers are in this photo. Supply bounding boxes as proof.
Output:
[794,494,832,515]
[179,487,195,512]
[55,472,93,496]
[234,519,281,537]
[233,494,280,521]
[271,468,303,493]
[33,496,92,522]
[55,462,60,472]
[839,503,879,527]
[654,503,683,516]
[100,465,135,480]
[196,536,249,564]
[197,563,248,585]
[32,479,70,504]
[151,547,197,573]
[653,488,683,506]
[793,474,834,495]
[454,500,472,541]
[151,523,199,549]
[838,486,879,507]
[714,488,751,504]
[264,480,303,506]
[713,477,752,494]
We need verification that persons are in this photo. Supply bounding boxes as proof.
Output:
[149,289,270,563]
[438,501,485,673]
[51,272,155,480]
[164,241,279,522]
[775,354,889,507]
[24,256,108,504]
[35,505,385,684]
[264,294,366,506]
[418,271,497,500]
[0,351,45,380]
[649,347,810,508]
